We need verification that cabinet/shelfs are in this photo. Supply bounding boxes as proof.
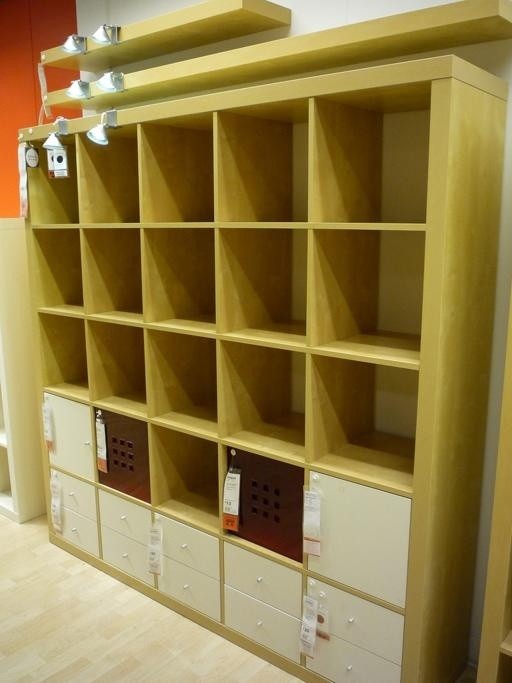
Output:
[20,56,470,678]
